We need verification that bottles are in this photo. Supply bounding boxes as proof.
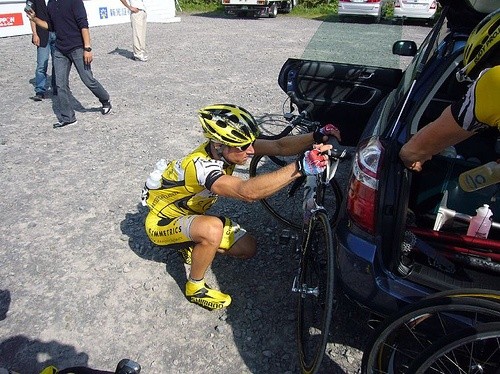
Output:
[302,173,319,212]
[154,159,169,173]
[467,203,493,239]
[303,193,316,224]
[142,170,162,204]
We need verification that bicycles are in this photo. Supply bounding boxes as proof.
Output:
[362,286,500,374]
[249,66,340,374]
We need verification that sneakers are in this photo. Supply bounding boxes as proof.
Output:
[181,245,194,264]
[186,280,232,309]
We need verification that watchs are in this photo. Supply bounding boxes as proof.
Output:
[84,47,92,52]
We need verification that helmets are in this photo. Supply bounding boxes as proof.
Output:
[456,7,500,81]
[197,103,260,146]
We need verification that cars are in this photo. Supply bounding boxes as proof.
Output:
[278,1,500,374]
[339,0,383,24]
[222,0,301,20]
[392,0,441,26]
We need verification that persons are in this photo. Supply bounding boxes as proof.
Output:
[120,0,149,61]
[26,0,58,101]
[401,9,500,171]
[26,0,111,127]
[142,104,341,310]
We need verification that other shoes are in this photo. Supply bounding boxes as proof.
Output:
[54,119,77,128]
[132,54,147,62]
[103,97,112,114]
[29,95,42,101]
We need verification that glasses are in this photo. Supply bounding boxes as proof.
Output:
[233,139,258,152]
